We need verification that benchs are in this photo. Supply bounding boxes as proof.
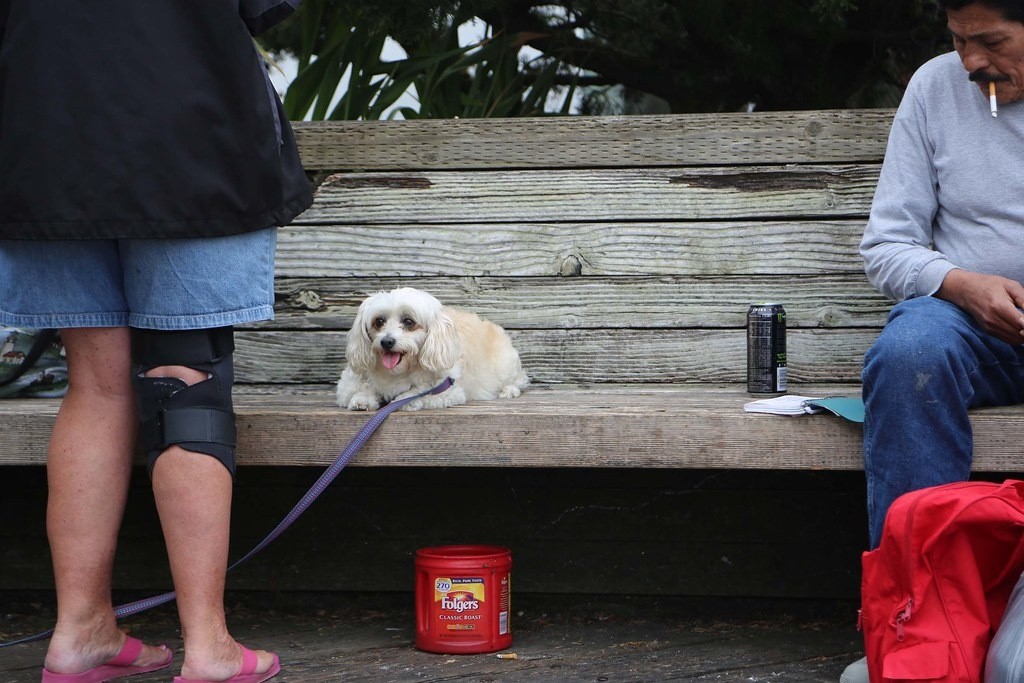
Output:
[0,111,1024,473]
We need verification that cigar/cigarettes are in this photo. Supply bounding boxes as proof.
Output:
[989,81,998,119]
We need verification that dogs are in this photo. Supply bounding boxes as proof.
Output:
[336,285,530,412]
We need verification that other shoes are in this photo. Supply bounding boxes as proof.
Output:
[840,656,870,683]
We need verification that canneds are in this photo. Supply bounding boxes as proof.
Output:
[746,303,788,397]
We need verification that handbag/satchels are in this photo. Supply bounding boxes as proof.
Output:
[856,479,1024,683]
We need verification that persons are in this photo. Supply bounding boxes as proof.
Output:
[1,1,309,683]
[842,0,1024,683]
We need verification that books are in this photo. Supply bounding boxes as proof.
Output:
[743,395,864,422]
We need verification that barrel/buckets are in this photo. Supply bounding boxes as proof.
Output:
[414,547,512,655]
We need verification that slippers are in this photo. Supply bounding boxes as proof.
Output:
[174,642,281,683]
[41,635,173,683]
